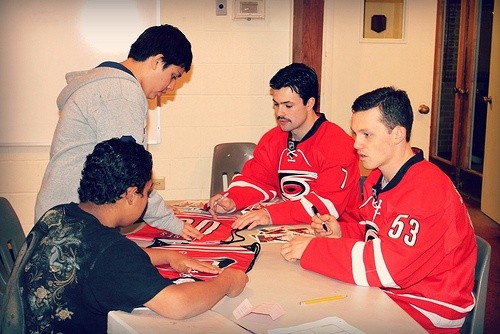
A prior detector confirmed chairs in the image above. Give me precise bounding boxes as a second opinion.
[460,235,491,334]
[210,142,257,199]
[0,197,27,305]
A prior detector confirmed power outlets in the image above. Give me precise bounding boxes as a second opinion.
[154,176,165,190]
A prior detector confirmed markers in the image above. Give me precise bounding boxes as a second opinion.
[311,206,329,232]
[209,192,230,212]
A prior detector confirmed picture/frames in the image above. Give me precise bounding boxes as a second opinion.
[358,0,408,43]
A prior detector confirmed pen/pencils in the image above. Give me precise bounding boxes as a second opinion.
[299,295,349,306]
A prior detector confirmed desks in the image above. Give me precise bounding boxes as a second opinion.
[109,189,429,334]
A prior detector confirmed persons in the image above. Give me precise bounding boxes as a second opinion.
[208,63,363,230]
[281,86,479,334]
[34,24,204,242]
[0,138,248,334]
